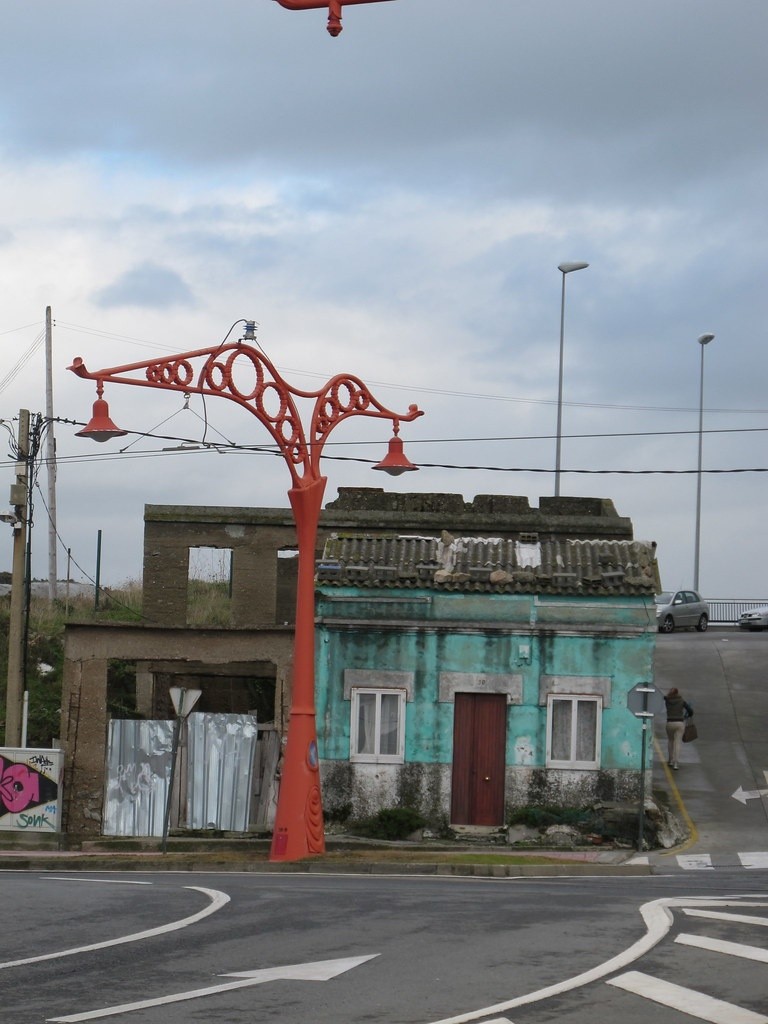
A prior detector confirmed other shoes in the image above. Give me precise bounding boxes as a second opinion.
[673,763,679,770]
[668,760,673,766]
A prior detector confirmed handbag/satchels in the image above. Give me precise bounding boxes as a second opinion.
[682,715,698,742]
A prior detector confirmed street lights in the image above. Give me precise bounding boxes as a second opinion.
[554,258,591,498]
[695,329,718,587]
[66,335,423,861]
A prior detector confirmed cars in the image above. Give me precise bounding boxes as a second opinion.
[736,606,768,634]
[652,590,710,631]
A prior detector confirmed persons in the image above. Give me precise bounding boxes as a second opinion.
[664,687,694,770]
